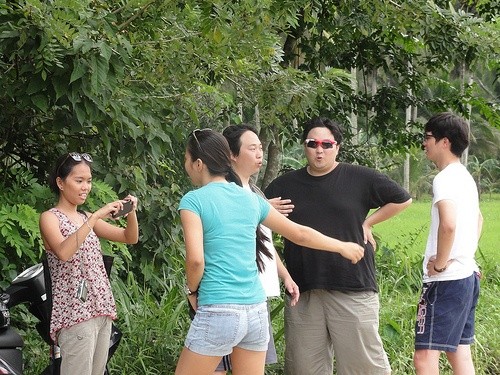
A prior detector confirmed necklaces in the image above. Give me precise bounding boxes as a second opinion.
[60,208,83,228]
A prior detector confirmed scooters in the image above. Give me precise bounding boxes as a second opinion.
[0,261,123,375]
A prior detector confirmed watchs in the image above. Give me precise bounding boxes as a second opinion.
[184,286,197,295]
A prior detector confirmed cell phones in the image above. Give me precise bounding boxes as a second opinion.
[111,200,133,218]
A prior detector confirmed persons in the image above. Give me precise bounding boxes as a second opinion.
[174,128,365,375]
[414,112,483,375]
[40,152,139,375]
[263,117,412,375]
[214,125,300,375]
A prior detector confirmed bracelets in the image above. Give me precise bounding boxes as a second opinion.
[434,264,446,273]
[87,222,91,230]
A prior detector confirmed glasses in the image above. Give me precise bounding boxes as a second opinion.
[57,152,93,178]
[305,139,337,149]
[423,133,435,140]
[189,128,213,154]
